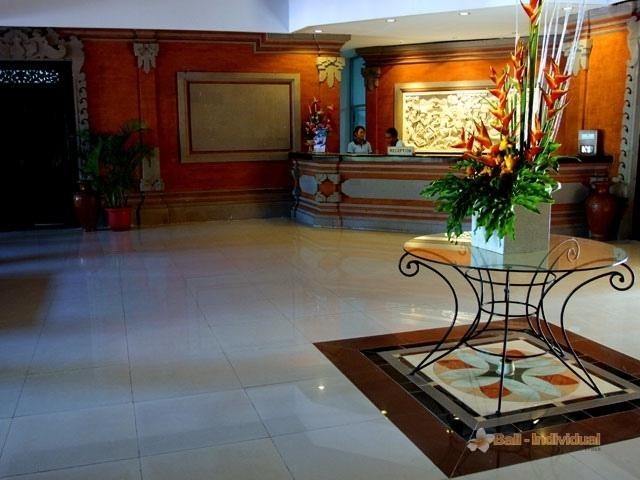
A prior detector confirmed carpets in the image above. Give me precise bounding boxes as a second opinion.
[311,315,639,477]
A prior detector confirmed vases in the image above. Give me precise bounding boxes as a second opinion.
[587,182,623,238]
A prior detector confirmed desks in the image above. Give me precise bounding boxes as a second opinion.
[397,234,633,415]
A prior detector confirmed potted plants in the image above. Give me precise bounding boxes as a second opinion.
[74,120,156,232]
[422,0,586,257]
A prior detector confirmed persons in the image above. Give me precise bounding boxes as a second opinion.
[384,128,406,154]
[348,126,373,154]
